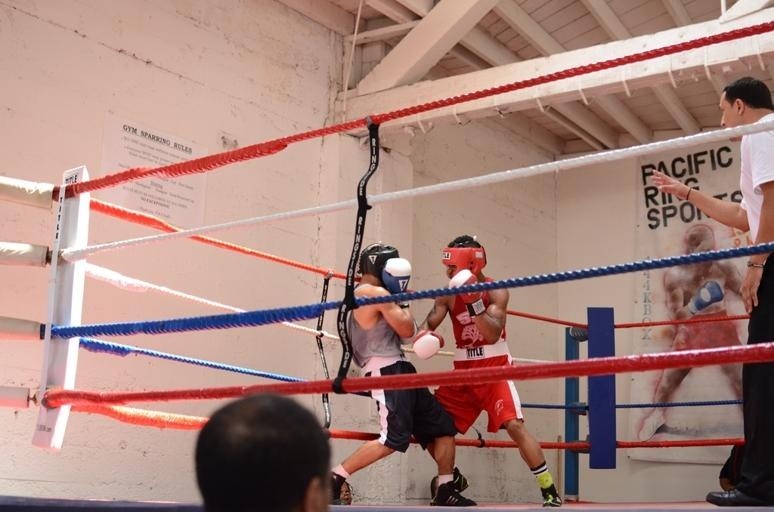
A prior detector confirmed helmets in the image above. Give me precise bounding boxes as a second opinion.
[441,235,486,278]
[360,244,399,276]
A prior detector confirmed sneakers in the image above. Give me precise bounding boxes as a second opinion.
[430,467,468,506]
[332,474,345,504]
[436,481,476,506]
[543,494,561,507]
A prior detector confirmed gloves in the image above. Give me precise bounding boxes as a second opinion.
[383,257,411,307]
[688,281,723,315]
[450,269,489,315]
[412,331,445,359]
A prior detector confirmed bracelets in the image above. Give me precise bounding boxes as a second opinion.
[748,262,765,269]
[685,186,692,202]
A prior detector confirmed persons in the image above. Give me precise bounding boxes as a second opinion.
[330,243,477,506]
[411,236,563,507]
[650,76,773,507]
[637,224,747,443]
[340,481,353,506]
[430,474,440,499]
[194,393,334,512]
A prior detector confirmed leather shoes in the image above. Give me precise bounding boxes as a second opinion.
[706,488,772,506]
